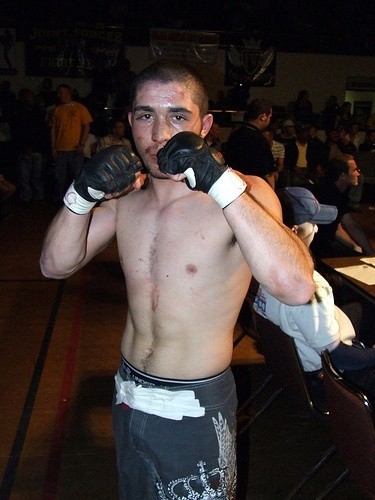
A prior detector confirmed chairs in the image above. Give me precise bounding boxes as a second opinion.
[277,347,375,500]
[233,296,260,349]
[235,319,330,440]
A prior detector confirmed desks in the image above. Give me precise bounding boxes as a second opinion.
[320,255,375,347]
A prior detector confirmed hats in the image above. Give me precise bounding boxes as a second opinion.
[275,186,339,227]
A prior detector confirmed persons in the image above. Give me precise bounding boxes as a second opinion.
[221,98,277,191]
[39,59,319,500]
[10,88,45,205]
[51,84,93,209]
[261,89,375,266]
[252,186,375,409]
[0,80,81,194]
[84,60,139,158]
[204,124,223,153]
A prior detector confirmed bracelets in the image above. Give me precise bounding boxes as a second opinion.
[79,142,85,147]
